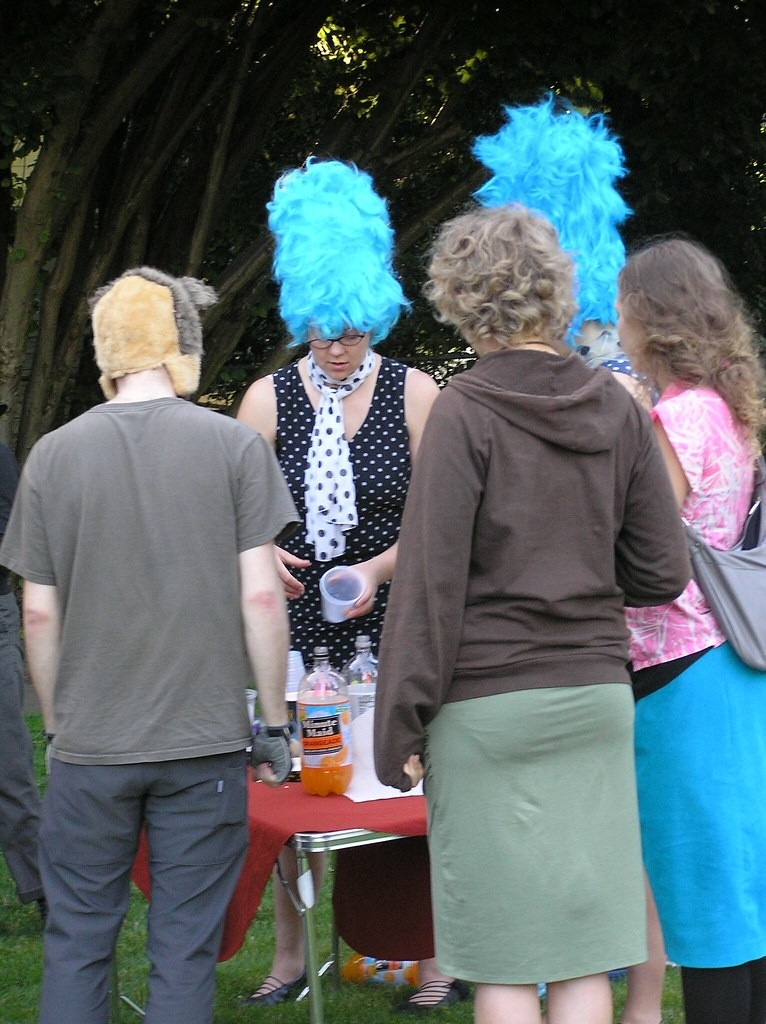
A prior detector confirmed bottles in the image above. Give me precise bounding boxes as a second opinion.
[296,646,354,796]
[329,952,420,988]
[341,635,379,724]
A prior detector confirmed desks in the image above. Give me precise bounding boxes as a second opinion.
[127,733,454,1023]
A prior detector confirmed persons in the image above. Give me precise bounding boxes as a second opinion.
[613,238,766,1024]
[1,265,304,1024]
[237,326,470,1013]
[373,205,693,1024]
[571,316,661,412]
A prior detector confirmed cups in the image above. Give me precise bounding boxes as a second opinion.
[285,650,306,702]
[318,566,367,624]
[244,689,258,725]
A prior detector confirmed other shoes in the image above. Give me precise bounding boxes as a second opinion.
[243,968,308,1004]
[36,896,47,920]
[395,979,470,1012]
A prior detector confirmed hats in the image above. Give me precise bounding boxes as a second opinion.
[0,404,9,418]
[88,266,220,401]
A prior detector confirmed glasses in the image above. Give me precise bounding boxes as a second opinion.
[306,332,365,350]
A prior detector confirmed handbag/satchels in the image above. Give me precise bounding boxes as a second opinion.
[680,454,766,671]
